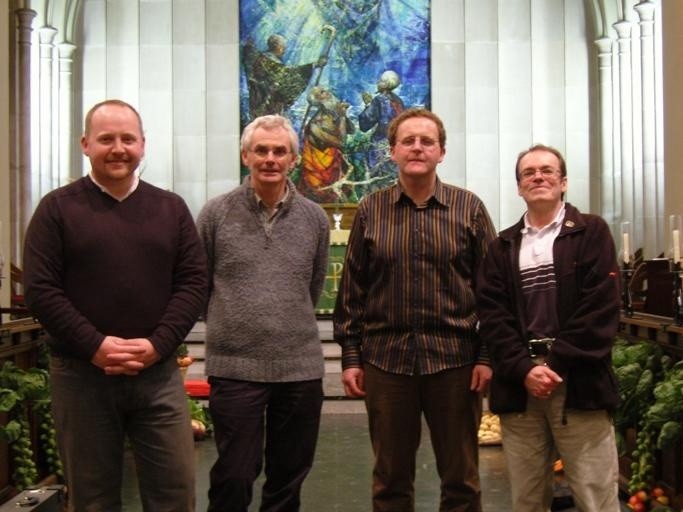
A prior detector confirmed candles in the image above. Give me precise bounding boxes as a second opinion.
[623,233,629,263]
[673,230,681,264]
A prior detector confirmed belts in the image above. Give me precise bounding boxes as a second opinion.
[529,337,559,360]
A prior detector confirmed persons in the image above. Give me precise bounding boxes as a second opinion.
[300,86,355,203]
[194,112,330,512]
[359,70,406,193]
[333,108,499,512]
[22,99,213,512]
[244,32,328,122]
[477,146,622,512]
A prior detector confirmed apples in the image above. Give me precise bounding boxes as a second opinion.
[629,487,669,511]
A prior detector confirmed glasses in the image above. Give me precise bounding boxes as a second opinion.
[518,166,562,179]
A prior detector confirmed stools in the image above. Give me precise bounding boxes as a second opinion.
[184,380,210,437]
